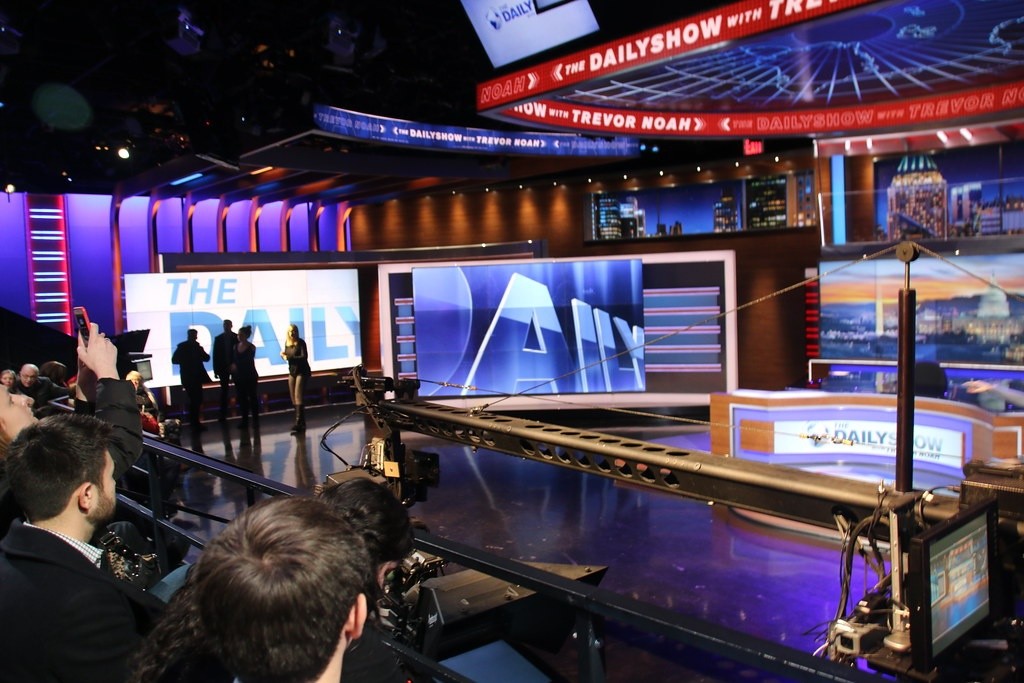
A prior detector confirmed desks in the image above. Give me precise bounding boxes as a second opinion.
[867,616,1024,683]
[710,390,1023,479]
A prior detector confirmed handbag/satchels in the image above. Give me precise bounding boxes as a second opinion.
[100,534,162,591]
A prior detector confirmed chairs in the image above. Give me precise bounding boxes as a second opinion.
[911,361,948,399]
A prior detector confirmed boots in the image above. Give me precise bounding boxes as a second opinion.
[290,404,306,432]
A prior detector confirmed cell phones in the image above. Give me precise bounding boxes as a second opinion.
[73,306,91,347]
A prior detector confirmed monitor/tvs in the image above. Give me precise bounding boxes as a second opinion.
[905,496,999,675]
[457,0,601,68]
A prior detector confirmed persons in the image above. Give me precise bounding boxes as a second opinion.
[0,323,165,591]
[227,325,260,430]
[0,362,182,445]
[192,498,374,683]
[128,476,416,683]
[172,330,211,432]
[213,320,239,421]
[280,324,312,432]
[0,415,170,683]
